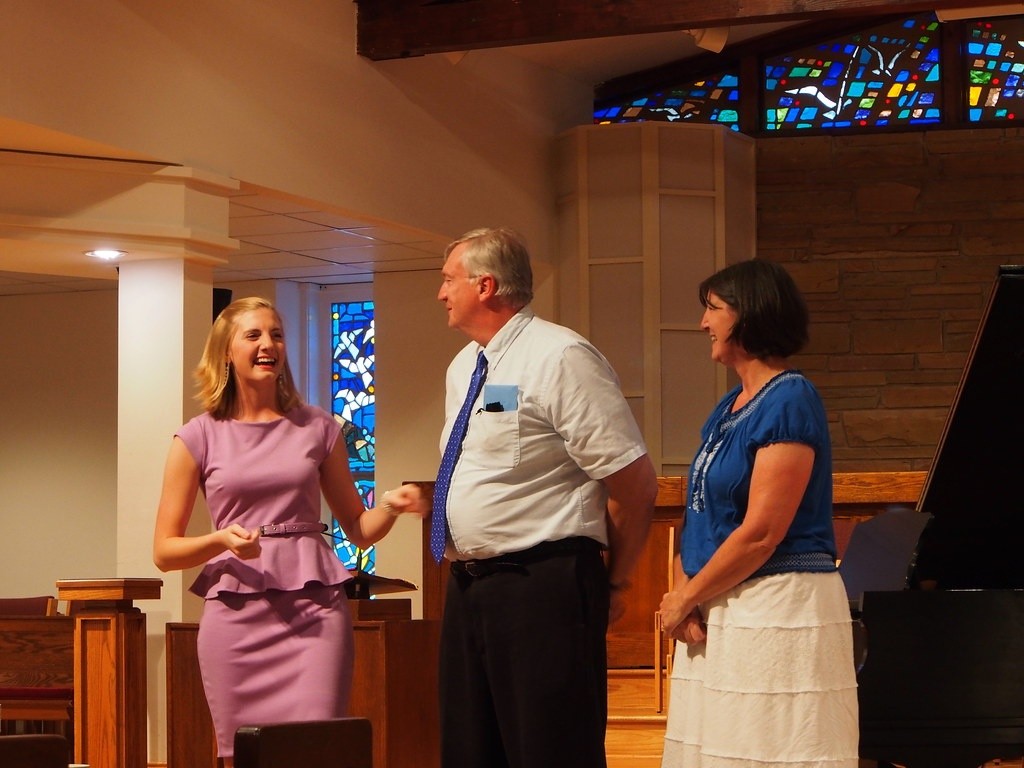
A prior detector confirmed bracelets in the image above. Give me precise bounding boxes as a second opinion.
[380,491,401,518]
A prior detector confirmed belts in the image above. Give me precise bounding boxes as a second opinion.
[448,536,599,584]
[247,523,328,537]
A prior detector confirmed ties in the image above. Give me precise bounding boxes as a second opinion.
[432,352,489,564]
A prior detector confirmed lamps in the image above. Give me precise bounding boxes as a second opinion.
[683,26,730,54]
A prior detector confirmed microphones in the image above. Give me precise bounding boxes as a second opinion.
[321,523,367,573]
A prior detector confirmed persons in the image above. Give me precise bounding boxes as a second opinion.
[660,255,861,768]
[153,297,433,768]
[435,227,658,768]
